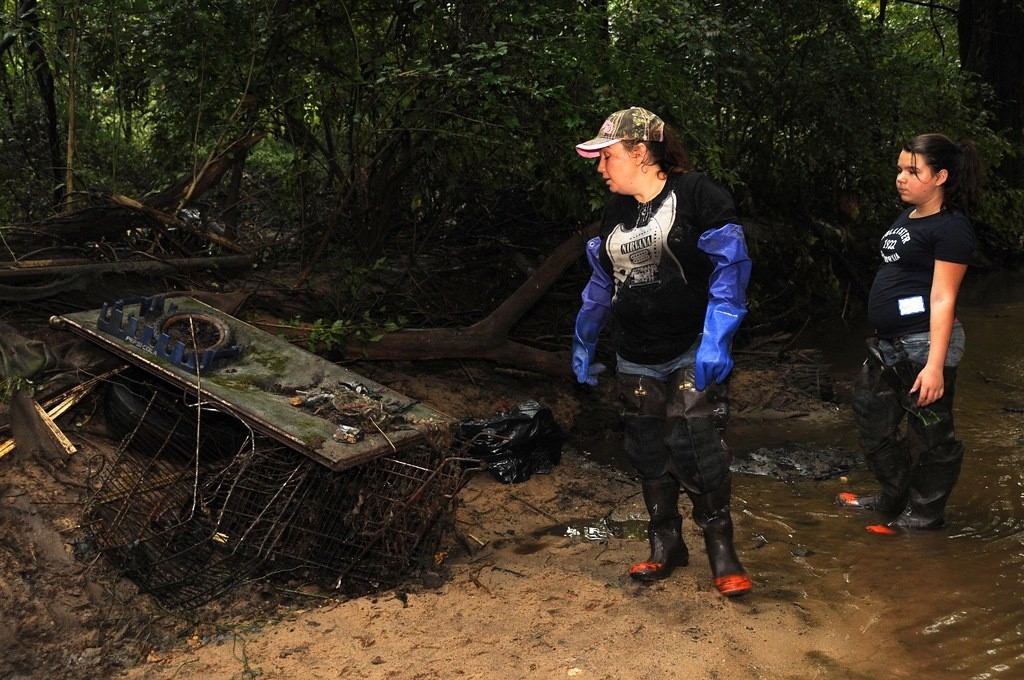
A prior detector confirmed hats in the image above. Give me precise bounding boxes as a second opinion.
[575,106,665,158]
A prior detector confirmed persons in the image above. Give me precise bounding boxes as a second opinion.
[570,105,751,598]
[834,133,975,535]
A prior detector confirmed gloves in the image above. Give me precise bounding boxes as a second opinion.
[695,223,752,392]
[571,235,612,387]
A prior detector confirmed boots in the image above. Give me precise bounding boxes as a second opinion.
[630,480,689,582]
[692,485,752,597]
[835,432,913,515]
[864,440,964,535]
